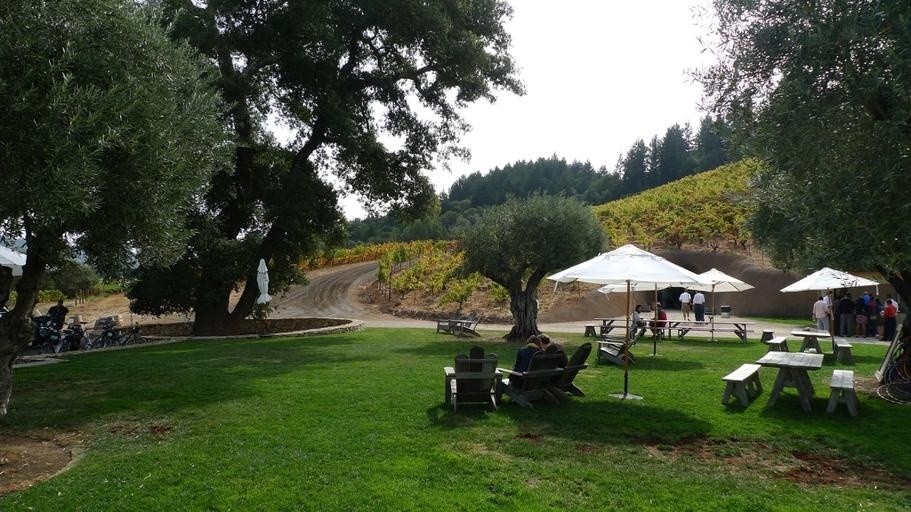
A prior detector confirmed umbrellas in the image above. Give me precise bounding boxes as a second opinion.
[256,258,272,334]
[779,266,880,294]
[597,282,671,319]
[683,267,756,329]
[545,244,699,340]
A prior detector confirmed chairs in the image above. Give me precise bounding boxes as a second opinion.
[434,310,485,338]
[595,323,646,366]
[496,350,565,409]
[554,343,592,396]
[442,353,503,413]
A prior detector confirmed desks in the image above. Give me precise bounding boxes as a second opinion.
[594,318,756,338]
[756,351,824,411]
[791,328,831,353]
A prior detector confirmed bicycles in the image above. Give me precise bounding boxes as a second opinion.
[31,317,150,357]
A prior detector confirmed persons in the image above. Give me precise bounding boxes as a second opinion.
[649,302,667,337]
[633,305,647,336]
[813,291,899,342]
[679,289,691,322]
[537,334,568,368]
[693,291,705,322]
[48,299,69,328]
[509,335,543,383]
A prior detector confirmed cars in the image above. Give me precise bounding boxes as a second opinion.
[0,306,11,318]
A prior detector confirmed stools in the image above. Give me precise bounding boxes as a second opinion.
[760,330,775,344]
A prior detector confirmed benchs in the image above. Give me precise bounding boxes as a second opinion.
[766,336,788,352]
[584,324,754,343]
[721,364,762,406]
[826,370,859,416]
[832,336,854,362]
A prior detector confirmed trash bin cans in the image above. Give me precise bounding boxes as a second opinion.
[720,305,732,318]
